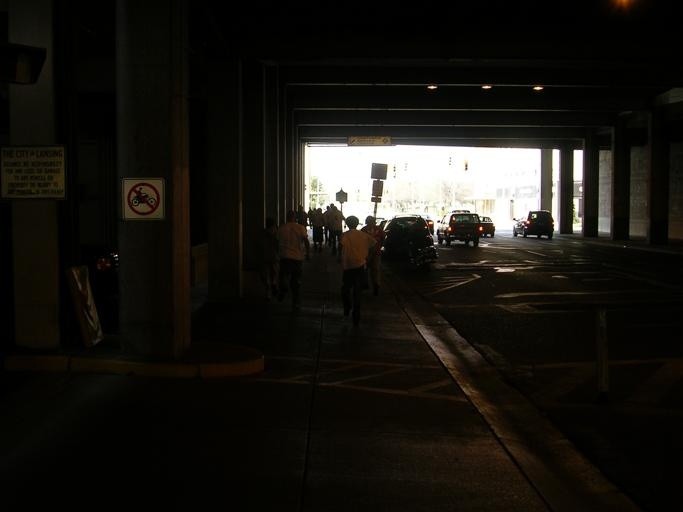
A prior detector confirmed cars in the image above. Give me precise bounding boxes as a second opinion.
[479,216,495,238]
[376,216,438,265]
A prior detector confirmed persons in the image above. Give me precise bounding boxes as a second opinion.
[275,204,387,329]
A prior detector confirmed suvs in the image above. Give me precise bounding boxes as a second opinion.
[437,209,481,247]
[513,210,554,240]
